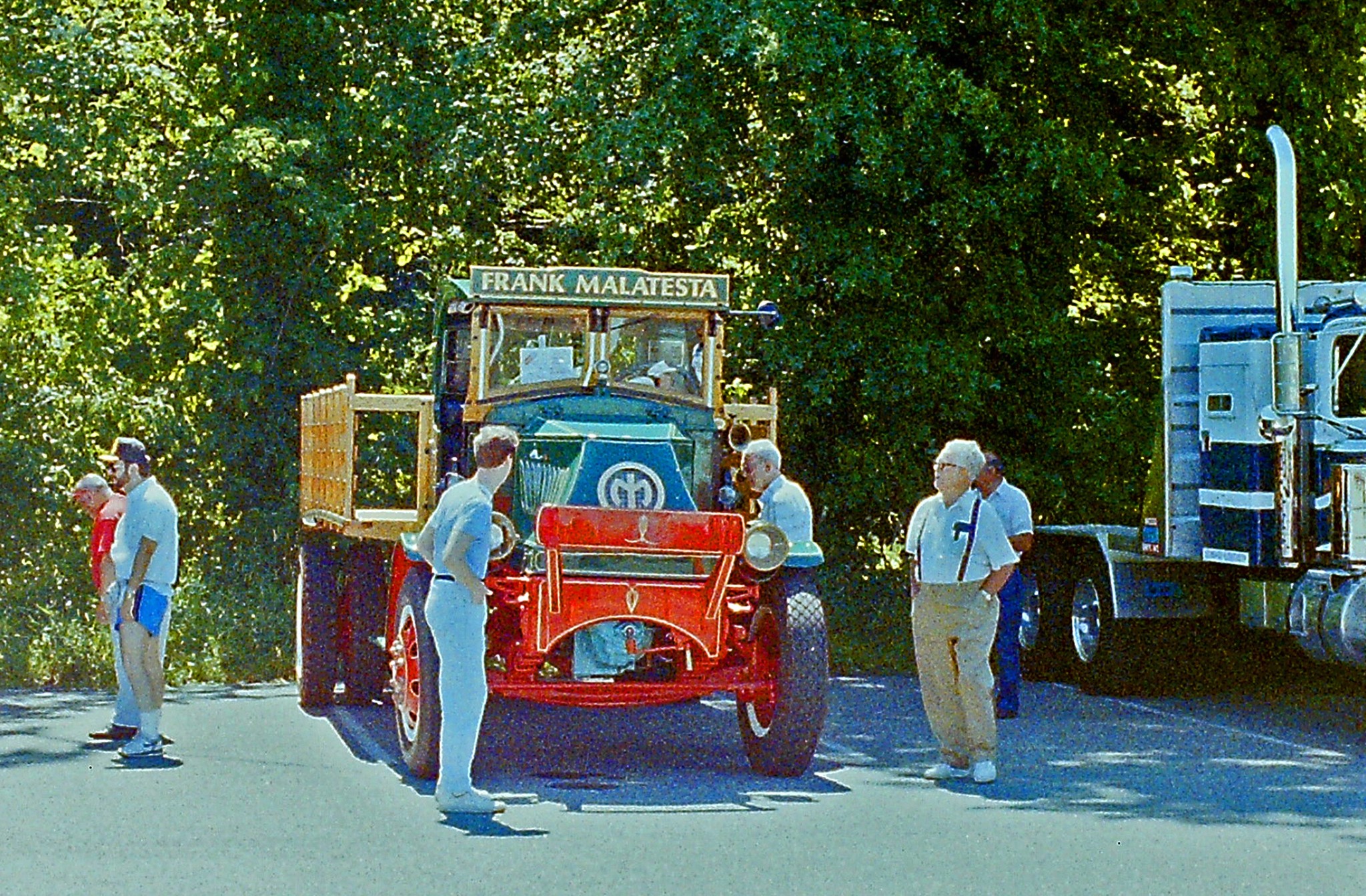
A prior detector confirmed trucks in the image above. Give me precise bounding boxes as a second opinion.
[289,263,832,782]
[1018,122,1365,698]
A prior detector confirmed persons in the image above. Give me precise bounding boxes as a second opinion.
[414,424,518,815]
[740,438,813,544]
[972,451,1033,720]
[75,473,172,742]
[905,439,1021,784]
[100,436,179,759]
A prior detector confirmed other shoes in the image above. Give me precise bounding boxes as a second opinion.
[118,736,164,759]
[924,763,970,779]
[973,761,997,782]
[437,788,506,814]
[89,723,136,740]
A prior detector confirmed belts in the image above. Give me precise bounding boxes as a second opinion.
[434,575,454,582]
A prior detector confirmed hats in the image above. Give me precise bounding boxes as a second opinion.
[98,437,148,464]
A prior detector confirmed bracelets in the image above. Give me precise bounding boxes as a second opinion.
[124,591,135,600]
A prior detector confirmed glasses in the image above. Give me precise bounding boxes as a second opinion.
[933,462,955,471]
[111,464,120,472]
